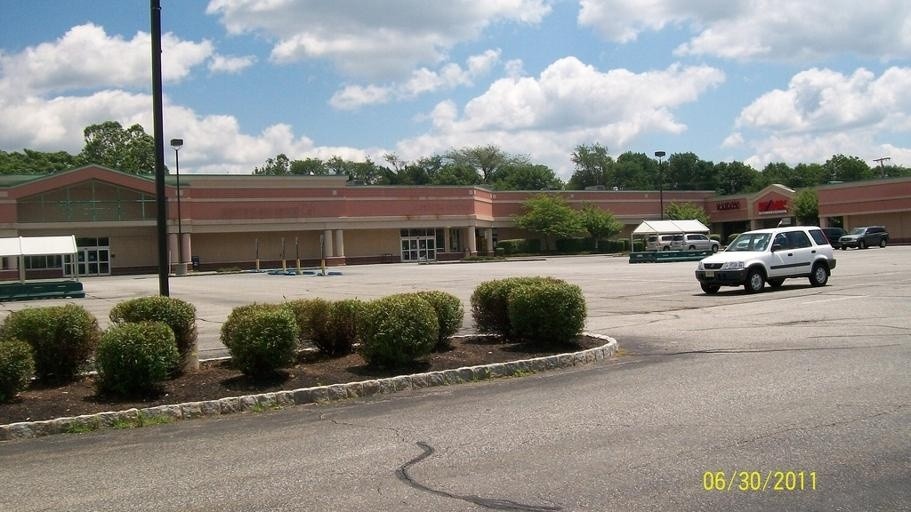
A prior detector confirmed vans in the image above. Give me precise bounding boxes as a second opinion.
[645,233,671,252]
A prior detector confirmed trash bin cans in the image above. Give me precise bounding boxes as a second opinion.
[496,247,504,257]
[464,247,471,257]
[384,253,392,263]
[192,256,199,270]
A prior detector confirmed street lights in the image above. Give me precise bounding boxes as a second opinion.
[171,139,184,264]
[656,151,666,220]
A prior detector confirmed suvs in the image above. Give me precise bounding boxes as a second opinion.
[669,233,722,253]
[696,226,837,293]
[839,225,889,249]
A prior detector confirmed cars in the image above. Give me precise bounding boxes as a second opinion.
[821,227,848,249]
